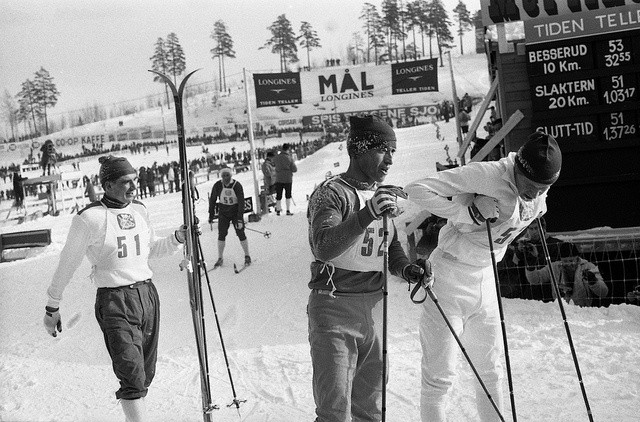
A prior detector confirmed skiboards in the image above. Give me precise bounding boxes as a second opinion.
[148,67,217,420]
[199,263,246,278]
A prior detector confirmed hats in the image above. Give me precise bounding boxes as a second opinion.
[98,154,136,181]
[221,165,232,177]
[515,131,562,184]
[560,242,579,258]
[348,116,397,158]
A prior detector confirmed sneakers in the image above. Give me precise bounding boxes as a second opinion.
[216,257,223,265]
[245,256,251,265]
[277,211,280,215]
[287,210,293,215]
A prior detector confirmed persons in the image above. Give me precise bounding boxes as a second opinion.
[307,116,435,422]
[207,167,251,269]
[519,245,610,310]
[404,132,563,422]
[84,163,181,204]
[437,89,505,169]
[262,150,276,206]
[11,166,28,209]
[43,154,201,421]
[514,219,578,264]
[276,143,296,217]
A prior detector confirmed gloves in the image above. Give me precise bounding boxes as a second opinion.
[365,184,408,219]
[525,251,537,271]
[402,258,435,289]
[235,220,243,229]
[582,269,598,285]
[468,194,500,226]
[44,306,62,336]
[175,216,203,244]
[209,215,214,223]
